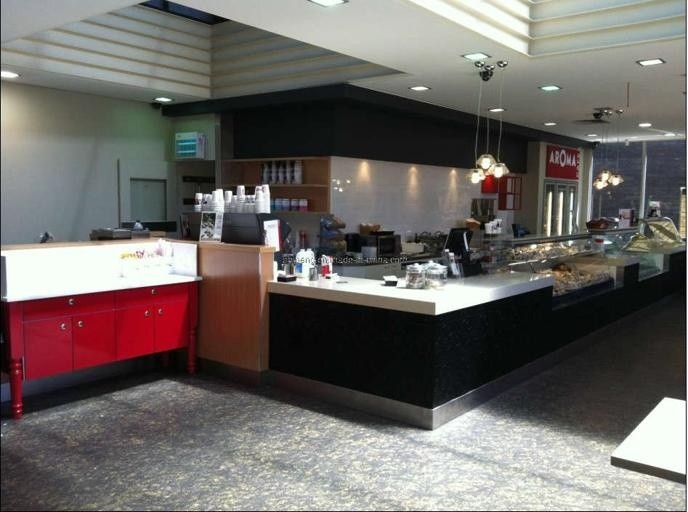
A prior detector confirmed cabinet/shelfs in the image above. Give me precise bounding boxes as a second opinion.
[0,283,203,419]
[221,156,331,215]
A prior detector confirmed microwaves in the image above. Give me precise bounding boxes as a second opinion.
[367,235,401,260]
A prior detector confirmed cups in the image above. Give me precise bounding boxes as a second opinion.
[270,198,309,213]
[321,264,333,279]
[194,183,270,214]
[485,217,503,233]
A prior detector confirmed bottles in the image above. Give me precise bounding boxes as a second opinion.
[405,259,449,289]
[295,248,315,278]
[133,220,142,230]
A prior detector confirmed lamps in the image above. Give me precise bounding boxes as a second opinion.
[592,107,624,191]
[466,60,509,184]
[174,130,205,161]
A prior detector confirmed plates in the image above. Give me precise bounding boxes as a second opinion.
[354,246,377,260]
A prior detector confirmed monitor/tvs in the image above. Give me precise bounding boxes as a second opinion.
[441,227,473,257]
